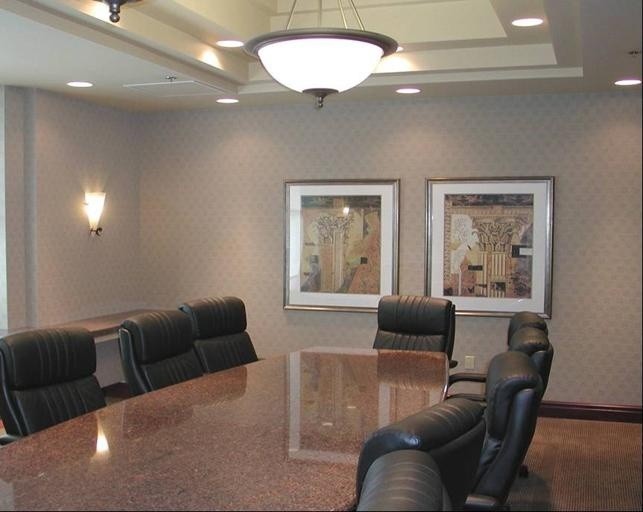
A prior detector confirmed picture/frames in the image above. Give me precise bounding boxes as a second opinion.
[424,176,552,320]
[282,178,399,313]
[285,346,391,468]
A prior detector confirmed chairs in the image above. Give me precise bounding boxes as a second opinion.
[177,296,258,375]
[358,398,487,511]
[117,311,204,398]
[506,325,554,390]
[449,349,546,511]
[444,310,549,401]
[370,297,459,371]
[0,326,107,444]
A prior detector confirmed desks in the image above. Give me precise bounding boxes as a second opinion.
[0,349,450,511]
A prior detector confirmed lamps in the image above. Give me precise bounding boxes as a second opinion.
[83,191,106,236]
[94,1,141,22]
[245,0,399,110]
